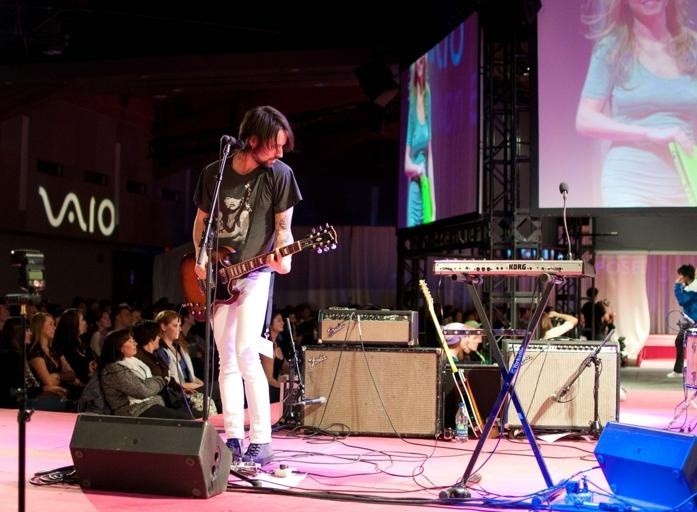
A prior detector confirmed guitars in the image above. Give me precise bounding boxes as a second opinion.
[180,222,338,322]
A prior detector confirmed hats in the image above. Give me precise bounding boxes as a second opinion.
[443,319,483,347]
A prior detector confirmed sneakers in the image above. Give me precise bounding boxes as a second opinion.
[242,443,274,465]
[223,439,243,461]
[666,371,683,379]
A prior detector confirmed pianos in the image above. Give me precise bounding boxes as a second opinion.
[432,257,596,278]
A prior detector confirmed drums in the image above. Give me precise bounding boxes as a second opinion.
[683,328,697,389]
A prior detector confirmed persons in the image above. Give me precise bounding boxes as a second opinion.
[571,0,696,208]
[667,262,696,381]
[349,285,614,421]
[400,51,439,230]
[192,105,303,469]
[2,296,318,423]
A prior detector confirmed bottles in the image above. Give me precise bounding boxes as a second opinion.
[455,403,469,443]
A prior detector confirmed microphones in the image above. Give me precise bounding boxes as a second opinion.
[559,182,574,261]
[222,134,245,150]
[299,397,326,406]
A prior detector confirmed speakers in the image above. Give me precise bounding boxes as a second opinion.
[502,340,618,436]
[70,413,233,498]
[445,365,500,437]
[319,312,418,346]
[301,345,441,439]
[595,421,697,510]
